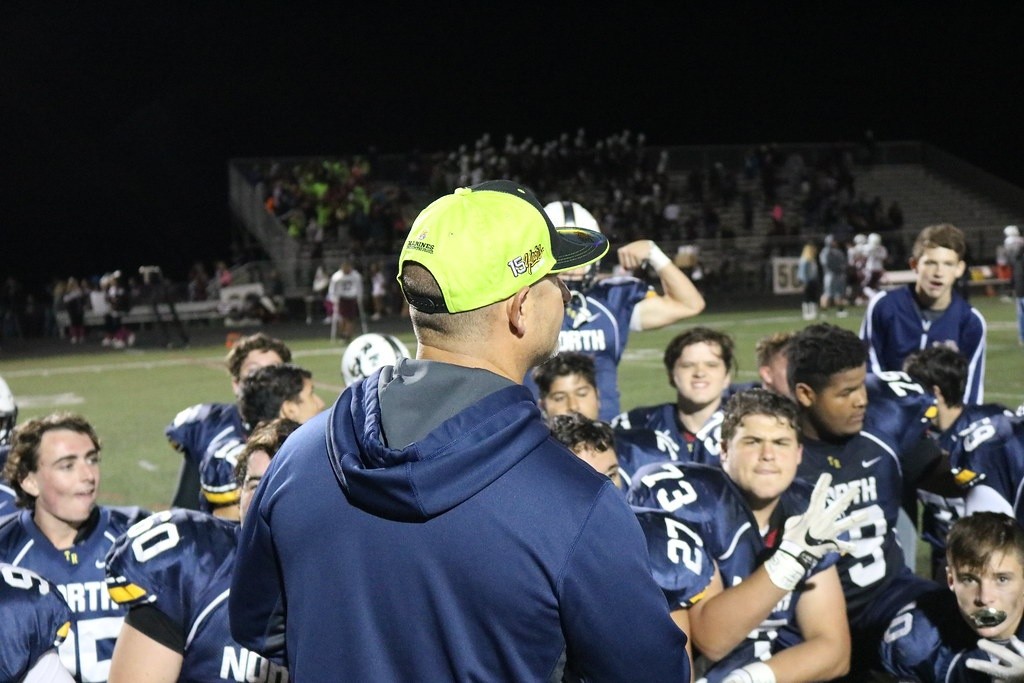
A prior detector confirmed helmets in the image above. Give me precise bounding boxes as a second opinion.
[542,198,602,297]
[0,376,17,452]
[824,235,839,247]
[869,233,882,244]
[855,235,867,245]
[340,333,412,390]
[1004,226,1020,236]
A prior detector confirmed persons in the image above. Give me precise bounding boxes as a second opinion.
[0,180,1024,683]
[0,127,1024,348]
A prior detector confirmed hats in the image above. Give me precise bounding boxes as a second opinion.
[397,178,610,315]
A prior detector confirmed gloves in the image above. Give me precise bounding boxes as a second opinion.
[764,470,867,587]
[966,635,1024,683]
